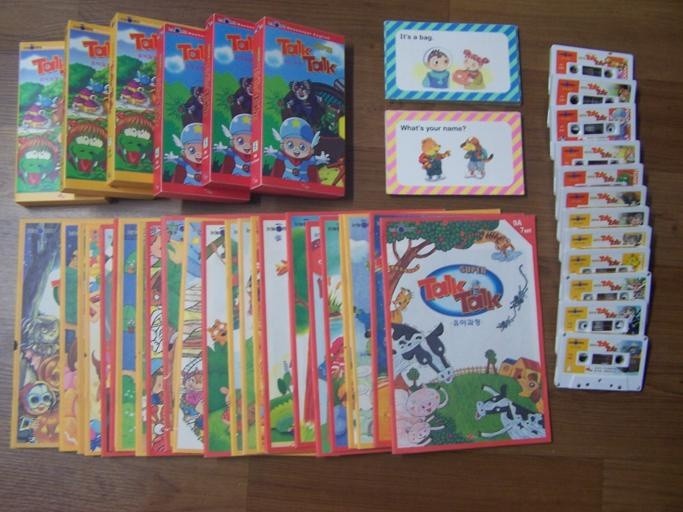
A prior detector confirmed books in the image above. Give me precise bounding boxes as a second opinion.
[11,208,554,461]
